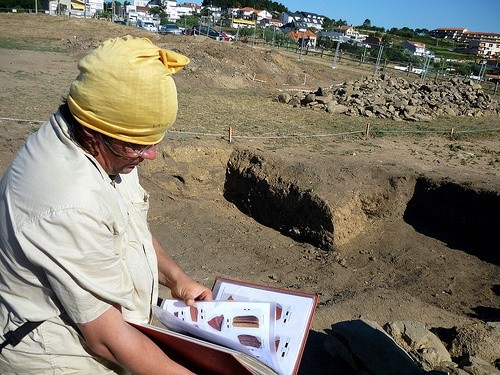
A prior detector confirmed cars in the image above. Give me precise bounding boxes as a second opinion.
[190,25,220,42]
[395,63,410,71]
[220,32,235,41]
[160,24,183,35]
[412,66,426,75]
[136,19,158,32]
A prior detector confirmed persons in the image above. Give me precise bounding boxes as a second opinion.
[0,34,215,375]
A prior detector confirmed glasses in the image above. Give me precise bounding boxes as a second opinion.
[128,143,153,158]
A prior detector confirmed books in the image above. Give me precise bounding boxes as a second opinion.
[122,275,318,375]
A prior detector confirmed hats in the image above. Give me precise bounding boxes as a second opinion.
[67,35,190,145]
